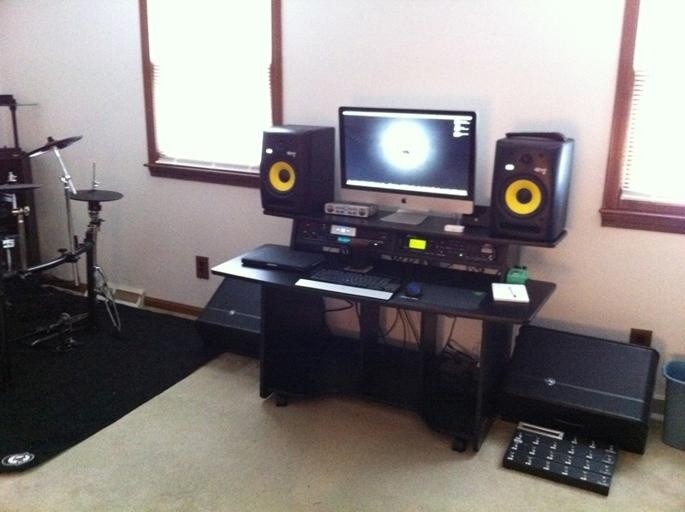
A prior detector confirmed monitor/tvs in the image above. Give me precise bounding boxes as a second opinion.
[338,106,476,226]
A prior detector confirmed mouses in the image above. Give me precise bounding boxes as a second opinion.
[405,280,423,296]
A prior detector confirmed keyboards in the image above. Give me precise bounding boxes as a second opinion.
[294,268,402,301]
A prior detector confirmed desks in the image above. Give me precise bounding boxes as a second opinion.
[210,211,555,450]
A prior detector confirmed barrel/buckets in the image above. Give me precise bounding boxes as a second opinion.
[660,361,685,449]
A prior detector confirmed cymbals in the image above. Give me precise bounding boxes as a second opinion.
[70,189,123,201]
[26,136,83,157]
[0,184,43,189]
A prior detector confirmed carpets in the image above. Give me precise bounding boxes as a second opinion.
[0,284,236,475]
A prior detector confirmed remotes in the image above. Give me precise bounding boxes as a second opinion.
[507,131,566,142]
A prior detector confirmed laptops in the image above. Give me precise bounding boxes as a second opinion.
[240,243,324,274]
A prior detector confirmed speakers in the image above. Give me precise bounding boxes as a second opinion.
[193,276,263,359]
[498,324,659,456]
[259,124,335,216]
[486,138,575,240]
